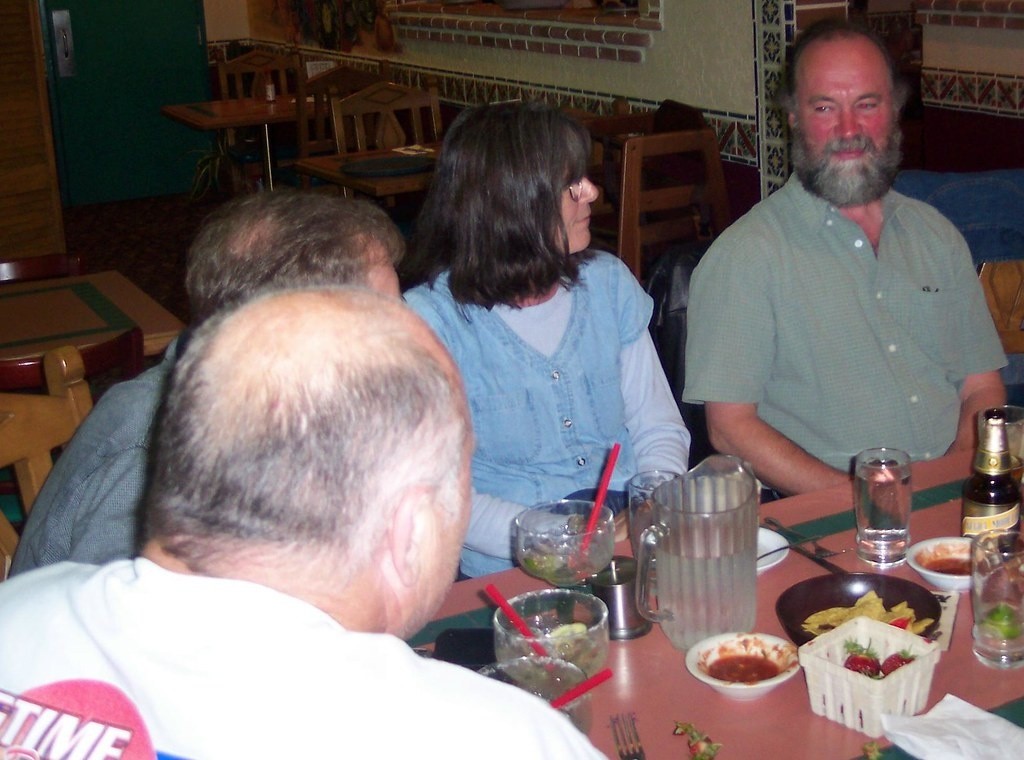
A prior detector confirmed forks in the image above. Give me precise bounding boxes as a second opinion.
[610,712,647,760]
[765,517,842,560]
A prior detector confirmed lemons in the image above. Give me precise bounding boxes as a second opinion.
[981,605,1023,638]
[547,623,588,641]
[524,555,561,575]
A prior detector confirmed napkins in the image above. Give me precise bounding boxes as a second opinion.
[879,691,1024,760]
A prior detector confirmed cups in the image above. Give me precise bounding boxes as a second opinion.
[514,498,616,588]
[474,654,593,736]
[969,529,1024,671]
[978,404,1024,459]
[627,468,683,559]
[854,448,912,570]
[589,567,652,640]
[492,587,611,688]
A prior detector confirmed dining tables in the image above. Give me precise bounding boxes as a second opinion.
[407,445,1024,760]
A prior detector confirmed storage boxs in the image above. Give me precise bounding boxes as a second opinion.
[799,614,941,741]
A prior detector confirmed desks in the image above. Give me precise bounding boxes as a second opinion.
[0,270,186,362]
[160,93,334,195]
[290,138,444,207]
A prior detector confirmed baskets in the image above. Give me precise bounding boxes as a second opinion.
[798,614,943,737]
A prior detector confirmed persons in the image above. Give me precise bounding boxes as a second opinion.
[6,191,407,578]
[680,18,1010,503]
[0,284,611,760]
[400,102,691,579]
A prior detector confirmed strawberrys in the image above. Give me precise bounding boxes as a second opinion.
[880,650,915,676]
[845,649,879,677]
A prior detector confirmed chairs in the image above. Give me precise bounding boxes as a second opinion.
[0,254,84,285]
[0,346,94,582]
[0,325,145,536]
[589,98,731,281]
[296,60,385,191]
[216,51,309,194]
[331,79,443,210]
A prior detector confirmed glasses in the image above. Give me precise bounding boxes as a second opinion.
[561,179,584,201]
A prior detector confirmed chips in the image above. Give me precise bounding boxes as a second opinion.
[800,589,935,636]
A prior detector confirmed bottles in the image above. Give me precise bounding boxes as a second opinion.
[959,407,1022,562]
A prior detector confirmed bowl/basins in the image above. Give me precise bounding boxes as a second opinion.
[756,527,789,576]
[775,572,942,647]
[685,632,801,702]
[906,535,1000,593]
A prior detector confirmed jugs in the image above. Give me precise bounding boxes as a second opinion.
[634,454,759,652]
